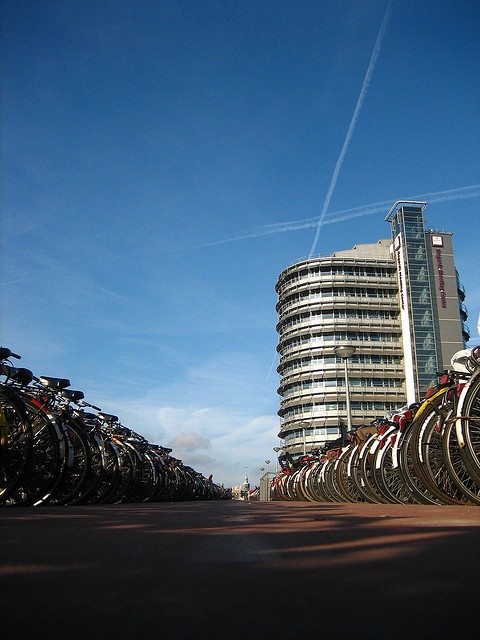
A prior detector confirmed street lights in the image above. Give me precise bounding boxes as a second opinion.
[260,467,265,474]
[265,460,271,473]
[298,421,309,454]
[332,345,356,433]
[273,446,280,474]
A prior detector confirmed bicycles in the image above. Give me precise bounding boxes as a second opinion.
[356,344,480,506]
[241,486,260,501]
[279,430,356,503]
[270,474,279,501]
[0,345,232,508]
